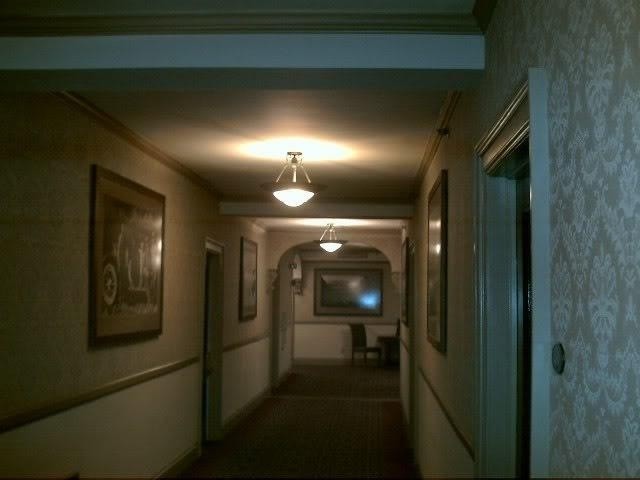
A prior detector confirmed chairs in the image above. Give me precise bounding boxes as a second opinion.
[349,324,381,367]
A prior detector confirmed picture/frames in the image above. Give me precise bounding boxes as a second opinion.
[400,238,409,327]
[313,268,384,316]
[427,169,447,354]
[240,236,257,321]
[90,165,165,348]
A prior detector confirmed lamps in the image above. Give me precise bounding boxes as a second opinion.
[314,224,348,253]
[260,152,327,207]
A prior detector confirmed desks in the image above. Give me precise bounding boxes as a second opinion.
[377,336,399,369]
